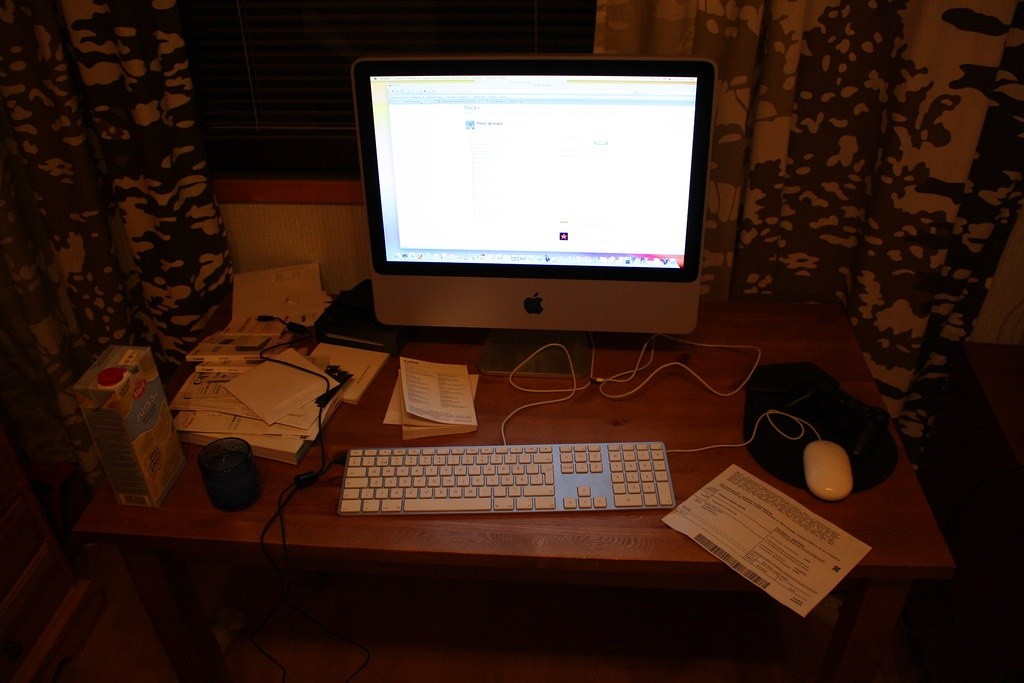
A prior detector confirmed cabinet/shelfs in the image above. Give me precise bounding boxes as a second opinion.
[0,413,104,683]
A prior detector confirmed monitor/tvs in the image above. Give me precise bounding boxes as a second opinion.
[351,54,718,377]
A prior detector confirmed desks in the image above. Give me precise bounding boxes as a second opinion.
[72,303,954,683]
[902,341,1024,683]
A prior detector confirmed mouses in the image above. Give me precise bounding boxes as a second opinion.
[803,440,853,501]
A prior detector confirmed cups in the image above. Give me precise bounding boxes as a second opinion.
[197,437,259,513]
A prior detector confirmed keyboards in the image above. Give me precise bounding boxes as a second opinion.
[338,441,675,515]
